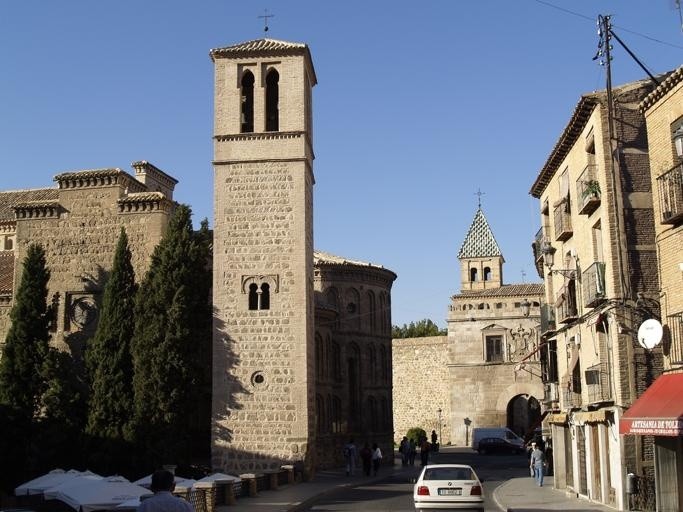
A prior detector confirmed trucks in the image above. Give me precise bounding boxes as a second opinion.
[471,426,523,452]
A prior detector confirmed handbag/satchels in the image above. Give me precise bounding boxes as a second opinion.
[530,467,535,477]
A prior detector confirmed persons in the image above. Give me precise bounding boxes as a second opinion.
[136,469,196,512]
[131,472,239,492]
[338,429,439,477]
[530,440,546,488]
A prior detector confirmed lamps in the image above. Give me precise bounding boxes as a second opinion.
[540,239,582,283]
[520,297,544,326]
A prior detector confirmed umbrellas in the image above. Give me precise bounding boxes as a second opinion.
[13,461,152,510]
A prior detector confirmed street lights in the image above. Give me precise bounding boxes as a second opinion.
[436,407,442,447]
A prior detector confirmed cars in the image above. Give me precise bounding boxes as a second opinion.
[411,464,484,512]
[478,436,520,455]
